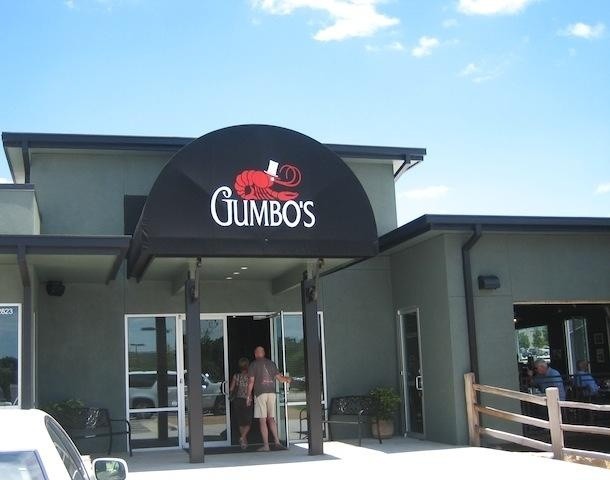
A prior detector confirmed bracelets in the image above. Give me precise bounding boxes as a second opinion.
[247,397,251,399]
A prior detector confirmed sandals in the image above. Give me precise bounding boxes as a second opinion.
[238,436,248,450]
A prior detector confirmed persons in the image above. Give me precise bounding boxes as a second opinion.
[230,357,254,449]
[574,359,599,394]
[525,359,566,431]
[246,345,292,452]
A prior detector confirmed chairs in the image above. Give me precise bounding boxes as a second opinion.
[569,384,602,440]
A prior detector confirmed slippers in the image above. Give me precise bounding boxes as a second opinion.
[255,447,270,452]
[274,443,286,450]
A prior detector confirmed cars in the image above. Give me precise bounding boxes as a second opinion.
[279,378,307,402]
[0,408,128,480]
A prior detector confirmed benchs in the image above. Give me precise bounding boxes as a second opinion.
[55,407,133,457]
[321,395,383,446]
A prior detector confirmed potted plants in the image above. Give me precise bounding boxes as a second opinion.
[368,384,402,439]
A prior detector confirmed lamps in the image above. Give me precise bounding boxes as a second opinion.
[46,281,65,297]
[477,275,500,290]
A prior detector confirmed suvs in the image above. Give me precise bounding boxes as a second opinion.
[129,371,226,419]
